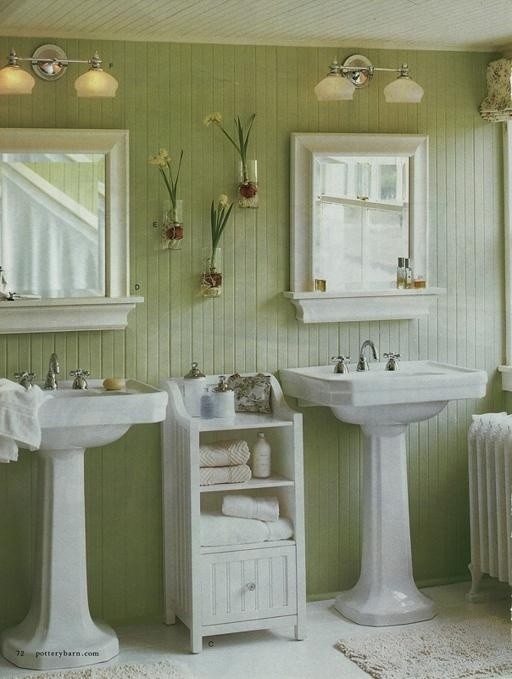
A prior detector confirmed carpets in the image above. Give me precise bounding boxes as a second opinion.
[8,659,193,679]
[336,612,512,679]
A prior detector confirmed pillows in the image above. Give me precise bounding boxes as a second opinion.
[200,511,294,547]
[0,378,53,464]
[222,494,279,521]
[199,439,250,467]
[200,464,251,486]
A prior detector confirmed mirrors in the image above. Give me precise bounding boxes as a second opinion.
[0,127,144,334]
[283,132,446,323]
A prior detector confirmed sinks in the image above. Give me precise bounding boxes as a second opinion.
[278,359,488,424]
[34,378,169,451]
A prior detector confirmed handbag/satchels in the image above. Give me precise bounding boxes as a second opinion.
[226,374,271,413]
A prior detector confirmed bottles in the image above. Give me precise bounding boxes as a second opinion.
[396,255,413,290]
[250,431,273,479]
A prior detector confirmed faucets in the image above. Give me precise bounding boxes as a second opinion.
[45,353,60,388]
[355,339,378,372]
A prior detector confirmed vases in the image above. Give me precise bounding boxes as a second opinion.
[163,200,183,249]
[201,248,222,298]
[238,161,259,208]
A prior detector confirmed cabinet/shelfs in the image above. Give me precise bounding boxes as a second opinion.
[161,373,306,653]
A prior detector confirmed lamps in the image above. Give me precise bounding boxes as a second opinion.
[314,54,424,103]
[0,43,119,97]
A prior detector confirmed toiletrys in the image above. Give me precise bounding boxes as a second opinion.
[405,257,412,289]
[397,256,405,290]
[413,275,426,289]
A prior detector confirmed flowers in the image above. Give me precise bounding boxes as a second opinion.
[149,147,185,227]
[210,195,234,274]
[203,113,257,185]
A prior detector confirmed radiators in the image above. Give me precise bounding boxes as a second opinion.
[463,411,512,603]
